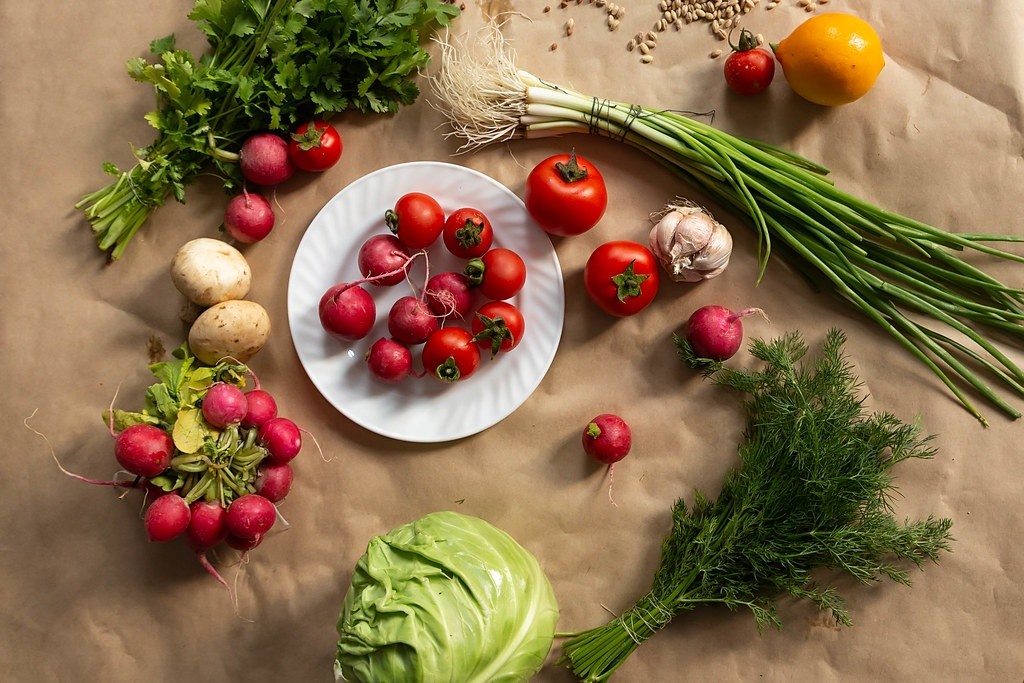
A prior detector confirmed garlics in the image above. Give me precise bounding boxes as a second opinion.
[647,196,733,282]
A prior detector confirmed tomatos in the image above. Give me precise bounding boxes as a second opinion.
[289,117,343,172]
[725,27,776,96]
[393,146,659,380]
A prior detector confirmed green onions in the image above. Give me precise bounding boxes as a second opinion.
[418,11,1024,427]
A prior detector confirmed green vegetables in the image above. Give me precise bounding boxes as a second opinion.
[72,0,459,261]
[555,329,954,683]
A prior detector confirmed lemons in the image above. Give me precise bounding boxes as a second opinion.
[769,14,885,106]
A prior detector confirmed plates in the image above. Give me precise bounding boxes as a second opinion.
[286,160,564,443]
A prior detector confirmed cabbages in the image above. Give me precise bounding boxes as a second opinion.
[335,512,559,682]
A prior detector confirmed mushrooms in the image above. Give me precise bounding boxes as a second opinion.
[169,237,271,363]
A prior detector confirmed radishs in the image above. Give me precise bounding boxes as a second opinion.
[22,353,333,608]
[225,187,274,243]
[320,235,470,383]
[239,132,293,186]
